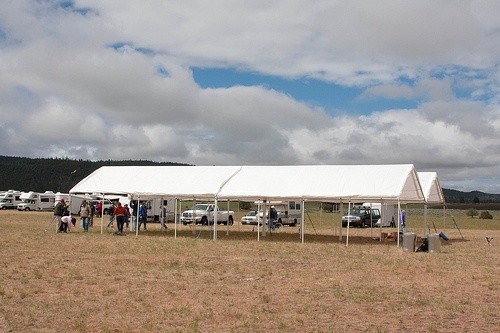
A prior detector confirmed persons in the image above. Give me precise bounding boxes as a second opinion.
[159,206,168,228]
[138,202,152,230]
[106,200,131,234]
[130,200,140,230]
[79,200,102,232]
[54,198,76,233]
[267,206,277,231]
[399,209,406,231]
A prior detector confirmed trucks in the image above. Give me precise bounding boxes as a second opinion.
[0,190,95,216]
[254,200,301,228]
[131,198,175,219]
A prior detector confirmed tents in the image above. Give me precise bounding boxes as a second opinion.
[130,171,445,236]
[69,164,425,248]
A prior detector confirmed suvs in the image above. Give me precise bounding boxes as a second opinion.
[342,206,380,228]
[93,200,115,216]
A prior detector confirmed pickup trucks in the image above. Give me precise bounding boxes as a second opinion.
[180,204,234,226]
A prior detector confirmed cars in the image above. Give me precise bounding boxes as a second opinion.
[241,211,258,225]
